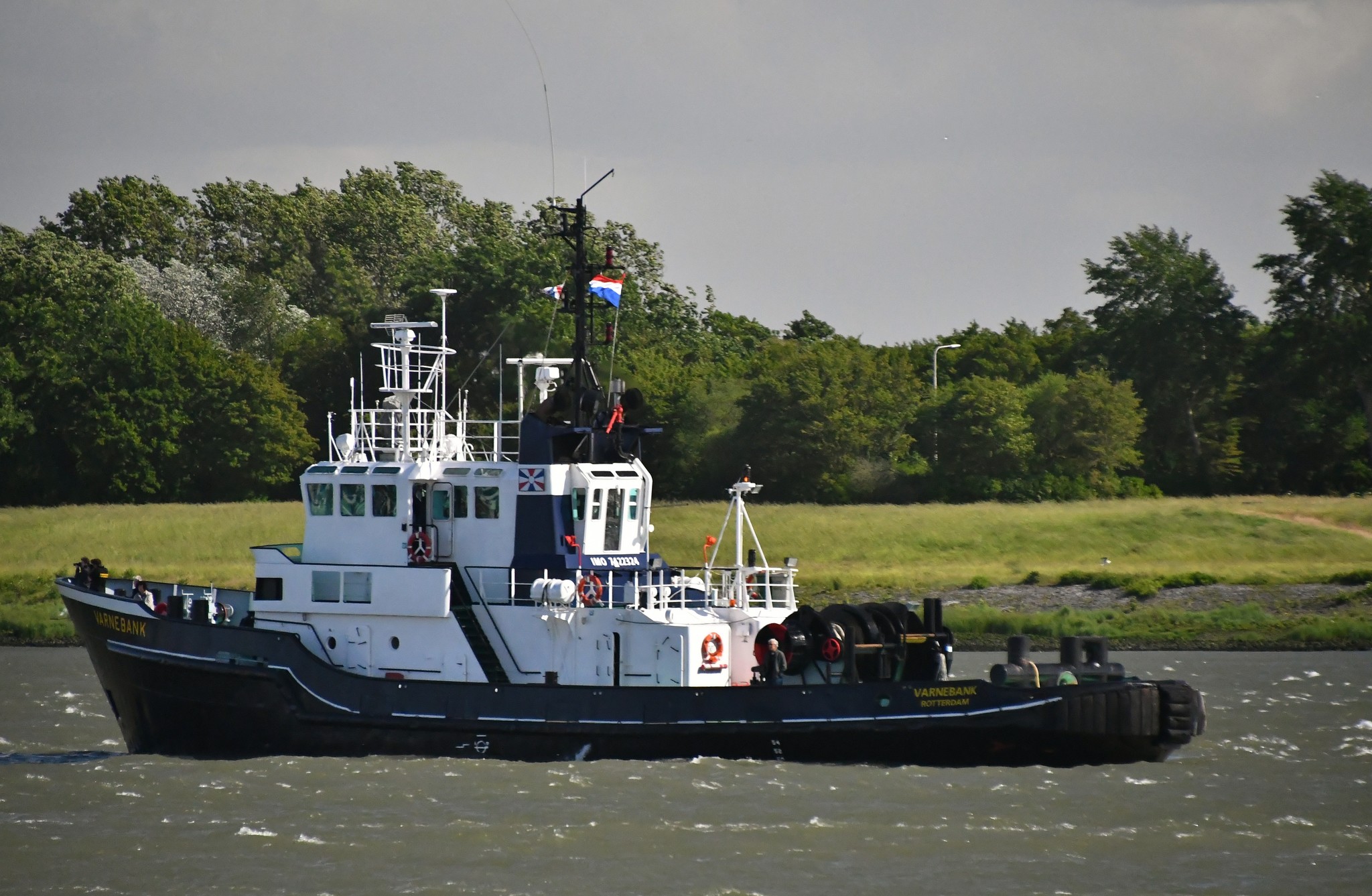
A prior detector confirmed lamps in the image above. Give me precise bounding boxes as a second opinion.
[751,485,764,494]
[741,463,752,482]
[784,557,798,568]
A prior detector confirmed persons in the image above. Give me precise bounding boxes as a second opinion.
[133,581,154,612]
[762,638,786,685]
[75,557,108,593]
[129,575,142,599]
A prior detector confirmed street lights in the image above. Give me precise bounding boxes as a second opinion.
[933,344,961,464]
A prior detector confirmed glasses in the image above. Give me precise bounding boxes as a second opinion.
[768,643,775,645]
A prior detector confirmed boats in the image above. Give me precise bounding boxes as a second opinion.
[51,163,1205,772]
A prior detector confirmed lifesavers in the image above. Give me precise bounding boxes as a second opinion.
[701,632,723,663]
[407,531,432,563]
[578,574,603,605]
[746,570,766,599]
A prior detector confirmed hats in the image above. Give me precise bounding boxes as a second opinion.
[135,576,142,581]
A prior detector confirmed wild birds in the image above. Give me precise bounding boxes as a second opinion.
[1098,556,1112,567]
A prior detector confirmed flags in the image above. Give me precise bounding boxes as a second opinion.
[589,274,622,308]
[540,284,564,300]
